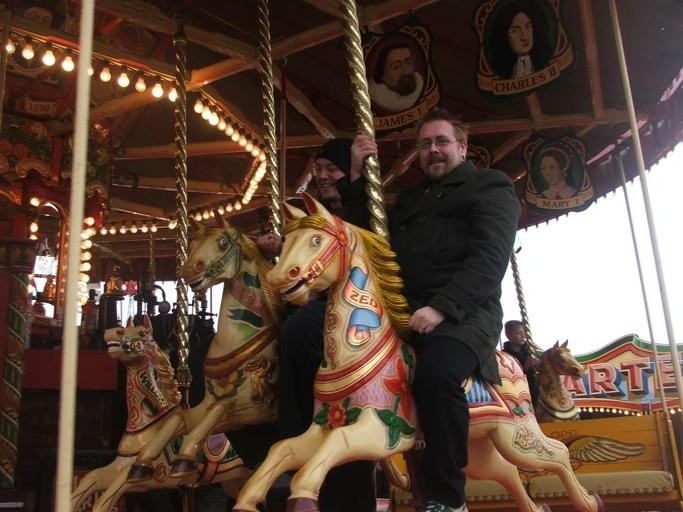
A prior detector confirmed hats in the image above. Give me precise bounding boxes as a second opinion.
[313,138,353,175]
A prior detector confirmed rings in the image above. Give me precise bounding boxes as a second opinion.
[267,236,273,239]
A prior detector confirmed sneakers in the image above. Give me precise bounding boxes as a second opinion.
[419,502,468,512]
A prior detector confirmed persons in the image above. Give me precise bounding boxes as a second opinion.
[536,144,581,198]
[153,302,174,348]
[486,2,556,81]
[334,105,524,510]
[366,41,427,119]
[187,314,215,409]
[501,319,542,412]
[255,136,359,438]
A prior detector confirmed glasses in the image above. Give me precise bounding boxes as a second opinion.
[414,140,457,149]
